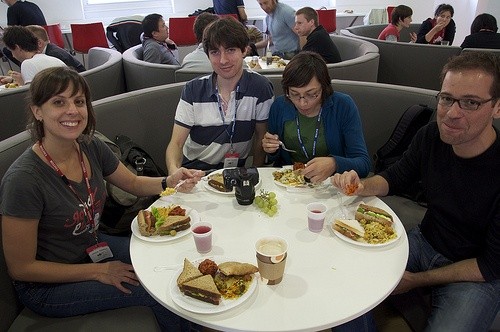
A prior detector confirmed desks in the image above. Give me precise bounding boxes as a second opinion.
[61,29,72,49]
[244,56,291,69]
[130,167,409,332]
[247,14,366,28]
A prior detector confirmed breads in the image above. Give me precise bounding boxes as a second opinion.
[198,259,218,276]
[293,162,305,170]
[218,261,259,276]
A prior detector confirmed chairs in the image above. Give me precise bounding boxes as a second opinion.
[366,8,386,24]
[70,22,115,66]
[45,23,76,56]
[316,9,337,33]
[168,16,197,46]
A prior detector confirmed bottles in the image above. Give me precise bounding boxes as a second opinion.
[252,56,258,65]
[267,49,272,65]
[235,172,256,205]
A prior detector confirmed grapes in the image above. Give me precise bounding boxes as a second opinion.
[255,191,278,216]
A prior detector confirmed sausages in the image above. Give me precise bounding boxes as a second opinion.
[208,179,226,192]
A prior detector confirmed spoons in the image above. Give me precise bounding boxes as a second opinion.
[161,169,201,201]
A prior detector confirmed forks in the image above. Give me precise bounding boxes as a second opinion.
[266,132,296,152]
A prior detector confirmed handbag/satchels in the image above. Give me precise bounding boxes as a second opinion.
[114,135,161,178]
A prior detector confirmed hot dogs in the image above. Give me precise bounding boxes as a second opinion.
[355,204,393,227]
[138,210,156,235]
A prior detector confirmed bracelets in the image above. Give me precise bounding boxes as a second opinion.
[162,177,167,191]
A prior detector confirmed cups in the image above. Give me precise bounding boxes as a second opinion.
[191,221,213,253]
[306,202,328,233]
[254,237,288,285]
[441,41,449,46]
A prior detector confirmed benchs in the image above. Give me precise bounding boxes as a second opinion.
[0,22,500,332]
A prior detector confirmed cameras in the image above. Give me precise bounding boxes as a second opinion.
[223,167,260,206]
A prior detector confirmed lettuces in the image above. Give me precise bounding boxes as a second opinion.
[151,206,165,227]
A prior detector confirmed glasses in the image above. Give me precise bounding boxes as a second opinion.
[285,87,322,100]
[435,91,498,110]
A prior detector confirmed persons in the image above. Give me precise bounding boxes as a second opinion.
[248,0,307,60]
[262,51,371,184]
[25,25,85,73]
[1,0,47,27]
[0,67,206,332]
[165,16,275,176]
[0,26,69,86]
[294,7,341,64]
[460,13,500,49]
[181,12,249,69]
[142,13,180,65]
[331,51,500,332]
[213,0,247,26]
[416,4,456,46]
[378,5,417,43]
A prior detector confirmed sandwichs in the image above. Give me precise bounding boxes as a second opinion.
[160,216,191,236]
[333,218,365,242]
[177,258,221,305]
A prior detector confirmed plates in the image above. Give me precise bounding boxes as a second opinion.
[131,203,201,243]
[273,165,330,189]
[170,255,258,314]
[202,167,261,195]
[328,206,402,247]
